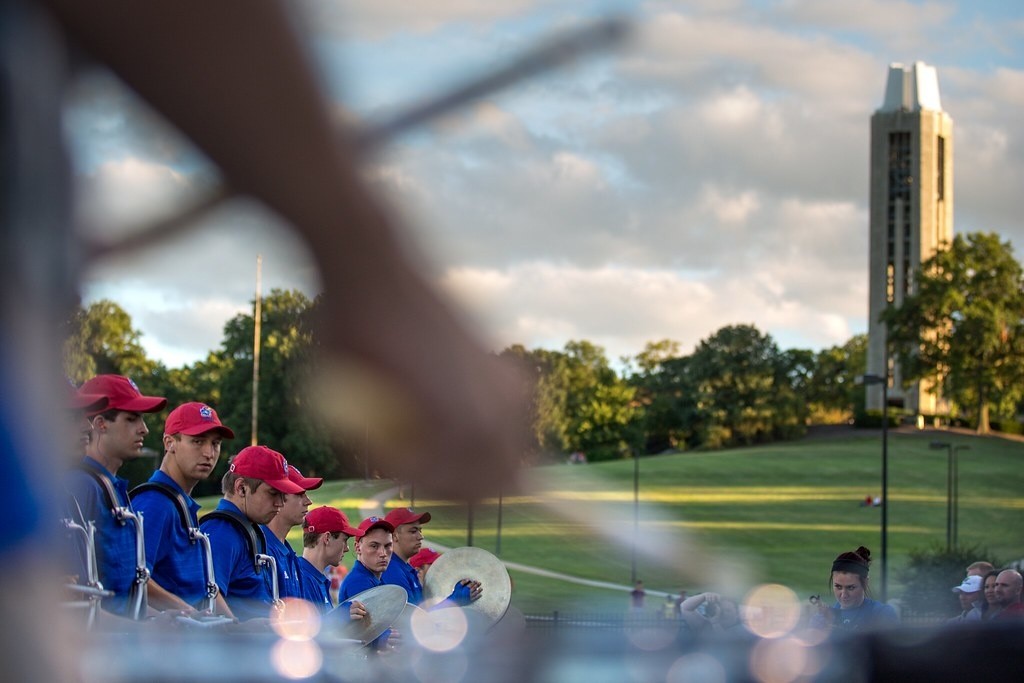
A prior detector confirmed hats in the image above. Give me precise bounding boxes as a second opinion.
[302,507,363,539]
[78,374,169,417]
[385,507,432,529]
[952,574,984,594]
[41,373,109,411]
[355,517,395,542]
[409,547,440,568]
[164,402,236,439]
[284,463,323,491]
[231,445,306,495]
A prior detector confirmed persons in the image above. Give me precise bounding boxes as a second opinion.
[858,494,872,508]
[661,590,687,618]
[47,374,214,636]
[127,403,239,634]
[873,496,881,507]
[408,547,442,588]
[808,545,900,683]
[945,561,1024,625]
[198,446,367,641]
[680,593,759,642]
[629,581,645,610]
[258,465,323,599]
[338,516,395,605]
[0,0,531,683]
[384,507,483,612]
[566,450,585,465]
[297,505,404,656]
[371,467,381,480]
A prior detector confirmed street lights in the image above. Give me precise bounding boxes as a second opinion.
[854,373,889,603]
[927,440,974,554]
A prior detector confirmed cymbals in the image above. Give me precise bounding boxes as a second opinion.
[421,548,512,629]
[360,603,430,665]
[323,584,408,656]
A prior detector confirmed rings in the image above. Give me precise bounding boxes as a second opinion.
[391,644,395,650]
